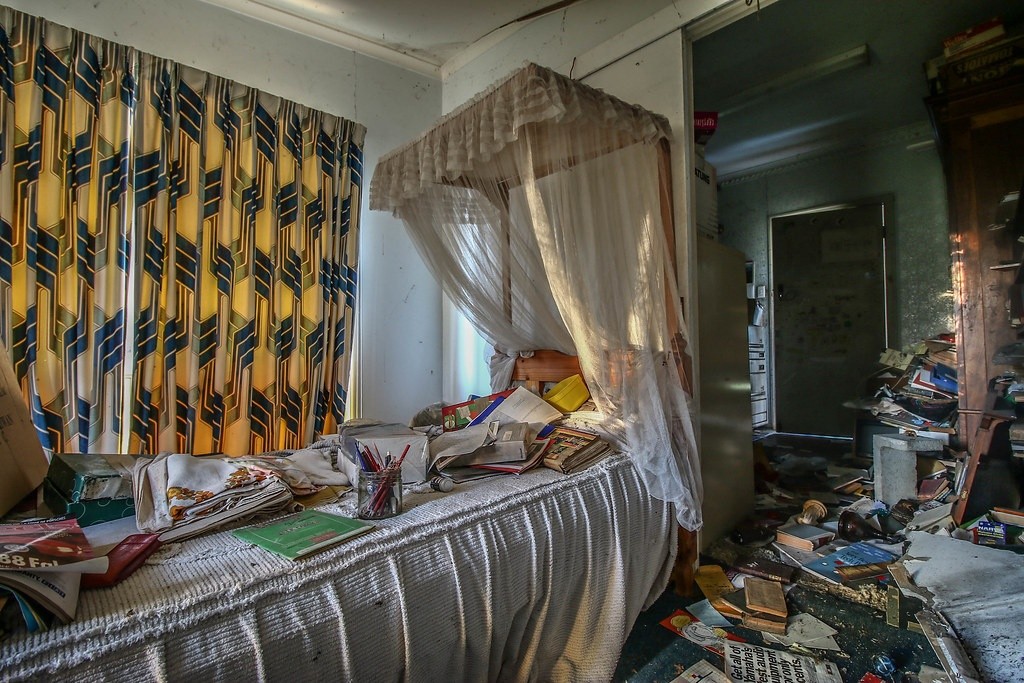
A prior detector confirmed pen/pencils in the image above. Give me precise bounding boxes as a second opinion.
[353,439,412,520]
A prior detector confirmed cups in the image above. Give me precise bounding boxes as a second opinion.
[357,468,403,519]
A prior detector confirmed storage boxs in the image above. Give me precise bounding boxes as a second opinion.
[447,422,530,467]
[542,374,590,412]
[337,422,429,489]
[922,17,1024,95]
[0,66,705,683]
[43,453,158,528]
[0,340,49,518]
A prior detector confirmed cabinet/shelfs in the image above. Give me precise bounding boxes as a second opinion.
[747,326,769,428]
[921,66,1024,449]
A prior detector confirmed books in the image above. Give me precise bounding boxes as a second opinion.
[0,510,110,639]
[677,326,1024,680]
[433,381,623,481]
